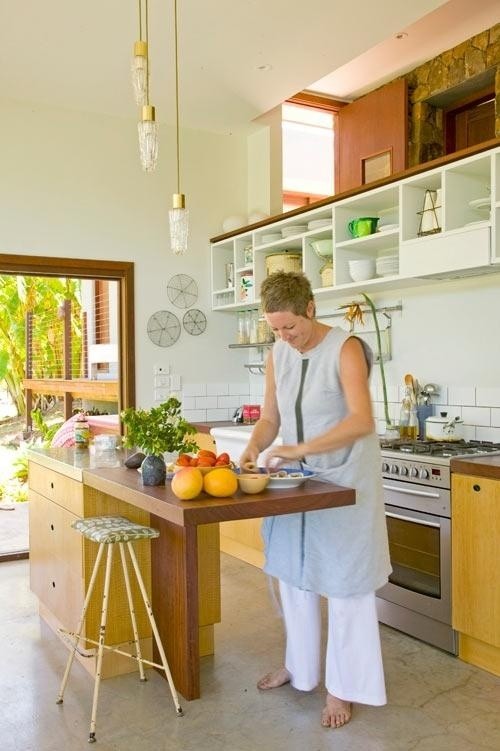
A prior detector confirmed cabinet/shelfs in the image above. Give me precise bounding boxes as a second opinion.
[210,147,500,316]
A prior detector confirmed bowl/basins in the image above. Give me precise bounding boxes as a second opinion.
[238,474,269,495]
[347,260,375,283]
[173,461,232,476]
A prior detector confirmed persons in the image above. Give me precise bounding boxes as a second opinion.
[237,269,396,729]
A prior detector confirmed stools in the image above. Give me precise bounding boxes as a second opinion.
[58,517,184,743]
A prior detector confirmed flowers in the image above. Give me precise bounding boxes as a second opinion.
[334,290,391,424]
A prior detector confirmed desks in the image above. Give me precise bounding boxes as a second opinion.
[26,431,358,705]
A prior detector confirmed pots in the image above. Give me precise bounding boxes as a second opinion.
[424,412,467,443]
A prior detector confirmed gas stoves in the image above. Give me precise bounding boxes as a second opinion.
[379,438,500,490]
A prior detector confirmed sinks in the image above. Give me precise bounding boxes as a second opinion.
[210,425,282,468]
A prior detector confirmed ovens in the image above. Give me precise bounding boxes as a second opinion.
[369,478,453,624]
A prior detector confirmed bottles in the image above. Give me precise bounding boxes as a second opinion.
[94,437,116,452]
[235,307,276,343]
[398,400,419,439]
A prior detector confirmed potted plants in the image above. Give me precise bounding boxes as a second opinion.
[120,400,199,487]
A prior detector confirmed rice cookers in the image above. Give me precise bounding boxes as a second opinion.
[263,249,301,277]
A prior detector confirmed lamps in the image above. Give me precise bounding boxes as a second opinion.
[168,0,189,256]
[130,0,158,176]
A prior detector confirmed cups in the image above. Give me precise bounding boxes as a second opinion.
[225,263,234,287]
[214,292,234,306]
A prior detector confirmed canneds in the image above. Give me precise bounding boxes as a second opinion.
[73,419,90,451]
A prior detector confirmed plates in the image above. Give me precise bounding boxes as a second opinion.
[378,223,399,233]
[137,463,176,480]
[470,198,491,211]
[258,217,332,242]
[465,219,488,226]
[232,467,316,491]
[374,256,399,277]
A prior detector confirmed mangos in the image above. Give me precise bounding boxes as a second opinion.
[178,450,229,467]
